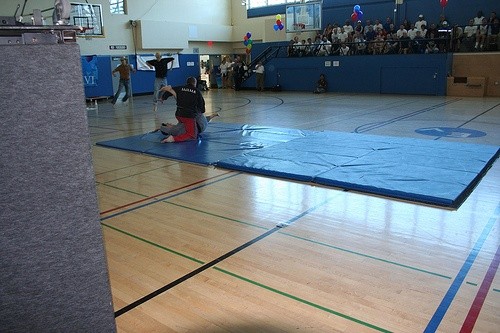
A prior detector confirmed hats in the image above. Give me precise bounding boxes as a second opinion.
[155,52,162,57]
[120,56,128,62]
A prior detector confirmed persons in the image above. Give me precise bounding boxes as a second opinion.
[160,77,205,143]
[255,61,265,91]
[148,85,221,141]
[317,74,327,92]
[221,56,250,91]
[289,11,500,54]
[146,52,175,112]
[111,57,136,108]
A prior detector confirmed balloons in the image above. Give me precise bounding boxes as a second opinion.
[440,0,447,7]
[243,32,253,56]
[351,5,363,21]
[273,14,284,31]
